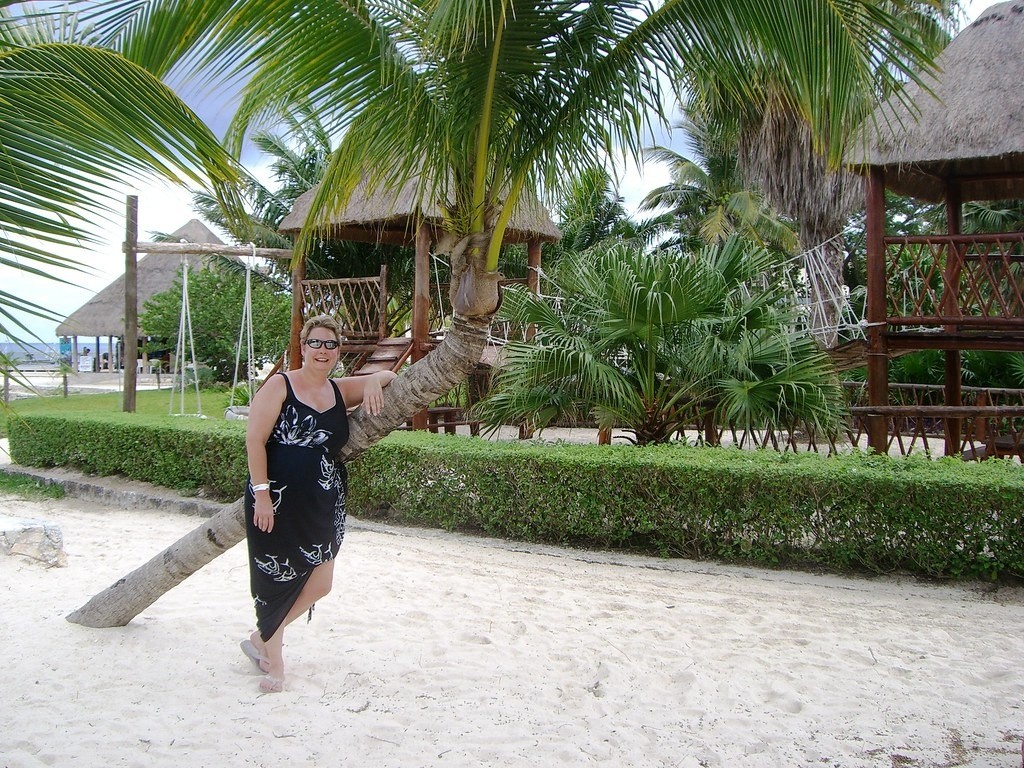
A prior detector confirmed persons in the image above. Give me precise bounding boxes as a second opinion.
[240,315,397,694]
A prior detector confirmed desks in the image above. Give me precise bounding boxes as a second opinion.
[405,407,463,434]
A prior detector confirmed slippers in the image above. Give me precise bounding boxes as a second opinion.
[259,674,286,693]
[240,639,271,675]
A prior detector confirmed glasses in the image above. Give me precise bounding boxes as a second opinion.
[304,338,339,350]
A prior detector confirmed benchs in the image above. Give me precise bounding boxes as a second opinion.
[398,421,485,437]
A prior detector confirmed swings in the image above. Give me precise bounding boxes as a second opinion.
[167,252,208,420]
[224,256,257,421]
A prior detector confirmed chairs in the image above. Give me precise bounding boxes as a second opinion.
[136,359,162,374]
[78,356,93,372]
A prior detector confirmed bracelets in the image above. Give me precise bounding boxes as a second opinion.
[252,484,270,492]
